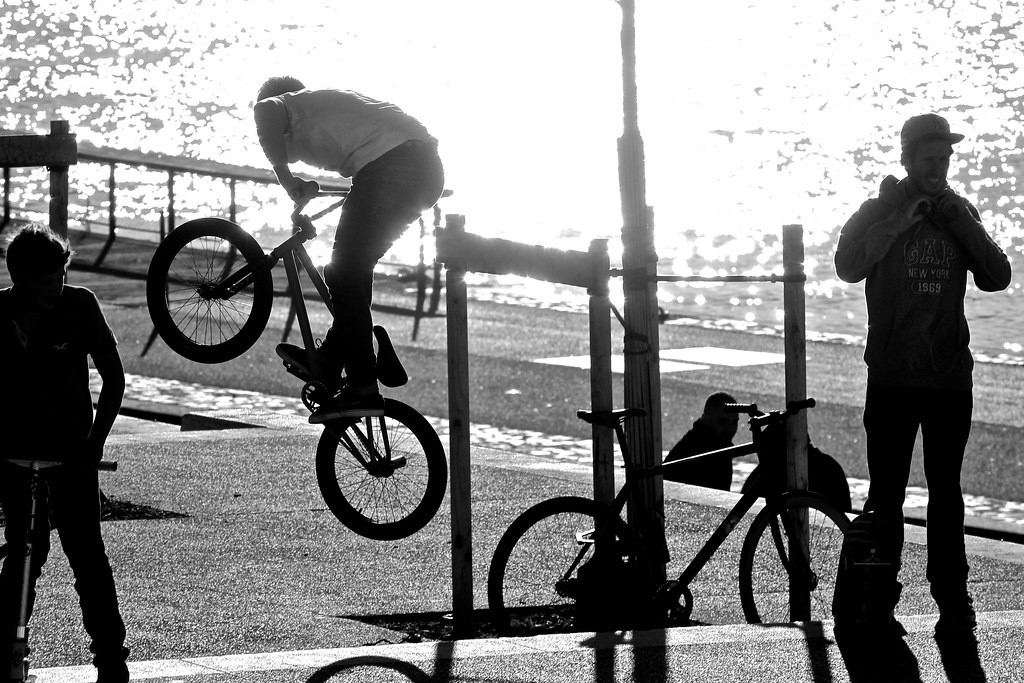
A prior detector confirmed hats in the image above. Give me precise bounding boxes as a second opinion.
[901,113,966,151]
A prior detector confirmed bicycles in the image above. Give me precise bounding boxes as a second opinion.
[147,185,447,540]
[488,399,850,637]
[0,456,117,683]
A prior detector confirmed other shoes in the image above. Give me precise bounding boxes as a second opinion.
[868,614,892,626]
[932,590,976,623]
[95,661,130,682]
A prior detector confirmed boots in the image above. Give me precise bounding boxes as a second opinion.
[275,322,350,388]
[308,353,386,423]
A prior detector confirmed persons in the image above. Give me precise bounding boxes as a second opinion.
[834,114,1012,629]
[254,76,444,423]
[0,225,130,683]
